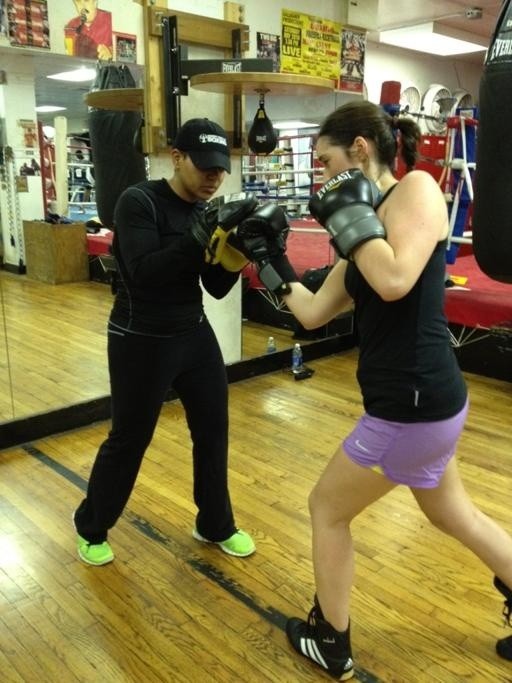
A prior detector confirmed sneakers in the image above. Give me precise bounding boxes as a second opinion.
[191,522,257,558]
[71,508,116,567]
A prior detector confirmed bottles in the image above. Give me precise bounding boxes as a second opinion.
[268,337,275,353]
[292,343,303,374]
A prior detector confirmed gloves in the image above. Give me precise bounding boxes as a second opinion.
[191,194,260,267]
[306,165,388,260]
[234,201,300,295]
[219,202,289,273]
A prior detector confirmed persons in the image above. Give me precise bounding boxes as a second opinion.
[232,99,510,680]
[64,0,114,60]
[75,117,257,566]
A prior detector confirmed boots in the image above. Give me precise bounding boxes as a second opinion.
[286,590,357,681]
[492,573,512,661]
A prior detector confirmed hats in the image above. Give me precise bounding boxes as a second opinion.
[173,116,232,174]
